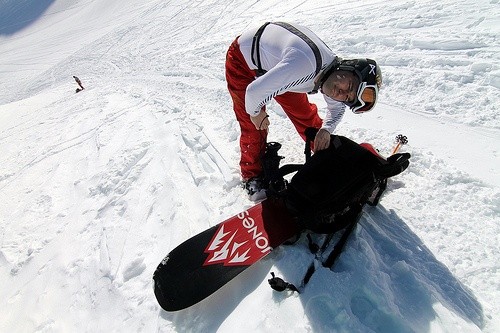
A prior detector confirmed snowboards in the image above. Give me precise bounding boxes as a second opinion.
[152,142,383,312]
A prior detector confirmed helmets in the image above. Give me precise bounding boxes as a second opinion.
[338,58,382,112]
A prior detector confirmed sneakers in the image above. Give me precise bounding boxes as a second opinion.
[243,176,267,201]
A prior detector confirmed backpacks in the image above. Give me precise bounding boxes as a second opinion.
[274,129,410,234]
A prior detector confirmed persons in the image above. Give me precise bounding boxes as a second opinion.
[225,21,382,202]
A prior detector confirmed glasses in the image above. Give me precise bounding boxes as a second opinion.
[352,58,377,114]
